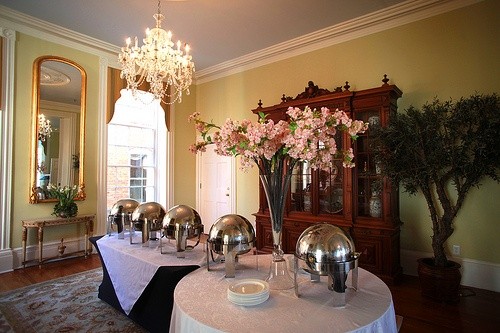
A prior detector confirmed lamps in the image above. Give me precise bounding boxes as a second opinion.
[37,113,53,142]
[117,0,196,104]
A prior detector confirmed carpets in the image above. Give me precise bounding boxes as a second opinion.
[0,267,404,333]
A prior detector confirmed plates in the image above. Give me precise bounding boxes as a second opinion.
[227,278,270,306]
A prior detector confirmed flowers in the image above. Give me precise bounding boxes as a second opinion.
[187,106,369,233]
[49,184,80,215]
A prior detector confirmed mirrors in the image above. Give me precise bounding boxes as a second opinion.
[30,56,87,203]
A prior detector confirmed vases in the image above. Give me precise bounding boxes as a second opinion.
[256,174,296,290]
[58,205,77,219]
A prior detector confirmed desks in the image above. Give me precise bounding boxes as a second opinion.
[21,213,96,270]
[88,231,226,333]
[169,253,399,333]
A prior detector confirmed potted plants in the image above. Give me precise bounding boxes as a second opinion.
[364,93,500,301]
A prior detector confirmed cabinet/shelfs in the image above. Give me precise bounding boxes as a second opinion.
[251,74,404,288]
